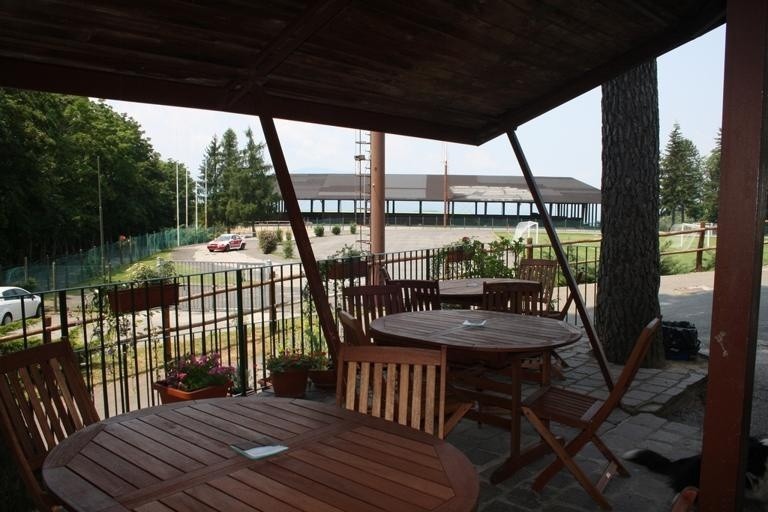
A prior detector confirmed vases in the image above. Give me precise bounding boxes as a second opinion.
[153,380,235,404]
[309,371,336,386]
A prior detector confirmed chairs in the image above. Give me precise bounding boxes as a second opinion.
[1,336,101,511]
[521,314,662,511]
[508,257,557,312]
[539,272,583,367]
[381,267,390,282]
[385,278,441,312]
[482,281,543,317]
[336,308,473,439]
[341,283,404,341]
[336,342,447,440]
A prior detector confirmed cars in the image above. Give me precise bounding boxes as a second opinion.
[0,287,42,324]
[207,234,245,252]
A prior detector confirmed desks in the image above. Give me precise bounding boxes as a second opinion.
[41,396,480,512]
[415,278,538,310]
[370,310,576,485]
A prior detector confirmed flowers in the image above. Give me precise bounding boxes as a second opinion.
[308,351,331,370]
[155,351,235,388]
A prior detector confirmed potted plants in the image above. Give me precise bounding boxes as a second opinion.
[87,264,180,377]
[430,236,475,281]
[302,245,368,351]
[266,347,311,398]
